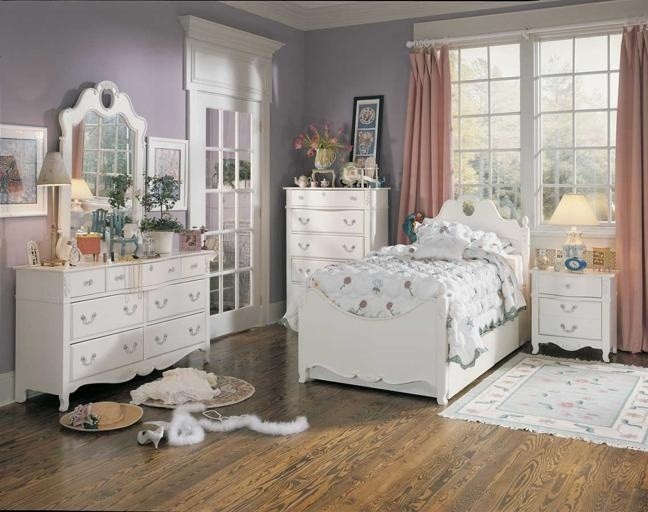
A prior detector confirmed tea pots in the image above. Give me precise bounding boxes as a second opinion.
[293,174,311,188]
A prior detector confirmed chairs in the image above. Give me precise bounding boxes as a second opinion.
[108,208,139,261]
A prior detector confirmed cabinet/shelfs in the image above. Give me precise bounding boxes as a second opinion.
[13,246,217,413]
[284,184,389,323]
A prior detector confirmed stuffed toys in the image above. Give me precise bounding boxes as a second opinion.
[403,209,424,243]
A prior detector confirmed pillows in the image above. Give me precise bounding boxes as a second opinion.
[413,217,510,261]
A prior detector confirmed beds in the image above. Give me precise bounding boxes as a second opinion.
[276,196,531,407]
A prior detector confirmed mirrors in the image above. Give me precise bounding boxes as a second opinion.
[56,79,149,260]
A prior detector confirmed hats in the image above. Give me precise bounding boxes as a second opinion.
[59,401,144,433]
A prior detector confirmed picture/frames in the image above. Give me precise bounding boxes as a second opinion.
[348,93,382,170]
[0,120,49,220]
[178,227,200,247]
[142,132,189,214]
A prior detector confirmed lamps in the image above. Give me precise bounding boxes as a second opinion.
[545,190,601,265]
[68,175,91,229]
[32,148,76,267]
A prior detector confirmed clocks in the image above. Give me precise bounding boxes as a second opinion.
[564,257,586,274]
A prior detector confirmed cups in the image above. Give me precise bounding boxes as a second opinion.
[309,178,329,188]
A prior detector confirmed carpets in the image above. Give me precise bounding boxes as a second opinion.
[435,349,646,453]
[136,371,255,414]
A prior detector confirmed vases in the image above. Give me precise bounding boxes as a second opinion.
[313,147,334,169]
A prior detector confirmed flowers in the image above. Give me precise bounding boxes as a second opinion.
[293,119,355,163]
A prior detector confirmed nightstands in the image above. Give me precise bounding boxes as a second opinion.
[526,262,621,367]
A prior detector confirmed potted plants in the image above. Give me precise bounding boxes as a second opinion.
[130,174,183,256]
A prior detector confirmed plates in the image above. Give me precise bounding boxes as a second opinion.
[340,162,360,185]
